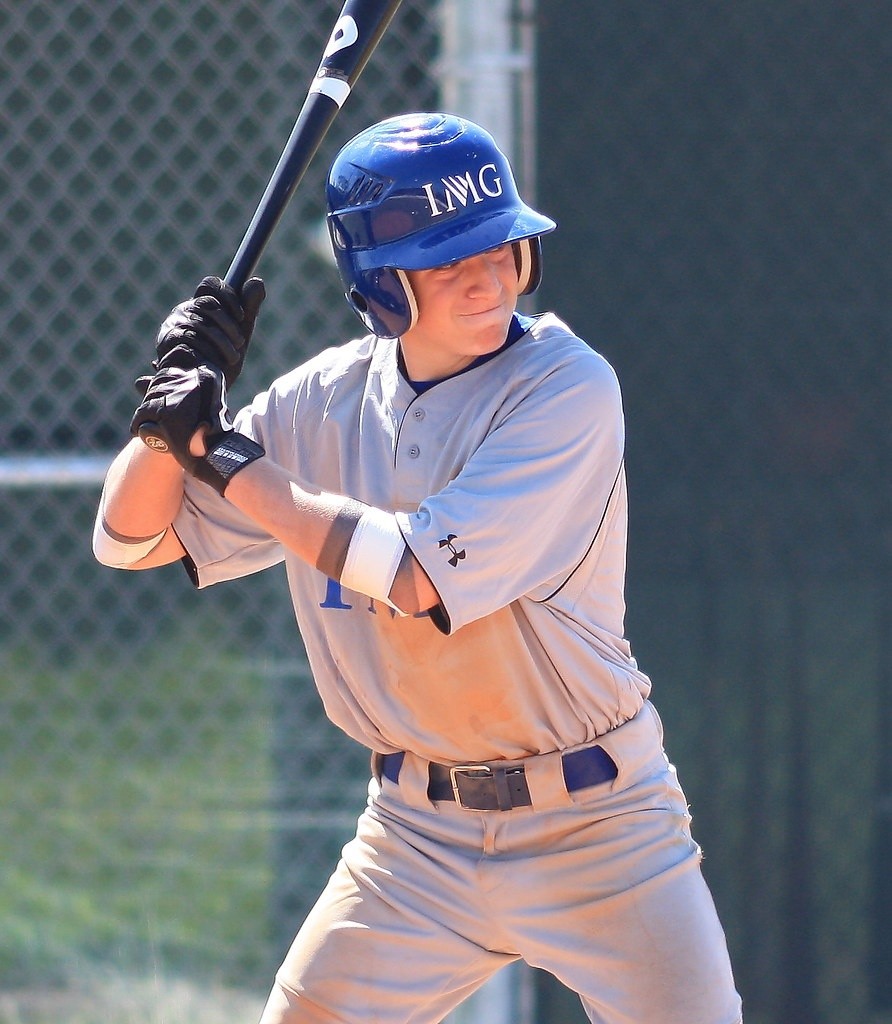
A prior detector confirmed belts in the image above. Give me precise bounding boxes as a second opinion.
[378,745,617,812]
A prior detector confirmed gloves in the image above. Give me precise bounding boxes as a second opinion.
[129,344,267,501]
[156,275,266,391]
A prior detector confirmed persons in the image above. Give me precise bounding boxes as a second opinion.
[92,114,743,1023]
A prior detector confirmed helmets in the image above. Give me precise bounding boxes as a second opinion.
[326,114,558,340]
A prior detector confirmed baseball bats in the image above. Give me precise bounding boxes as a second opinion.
[138,1,402,451]
